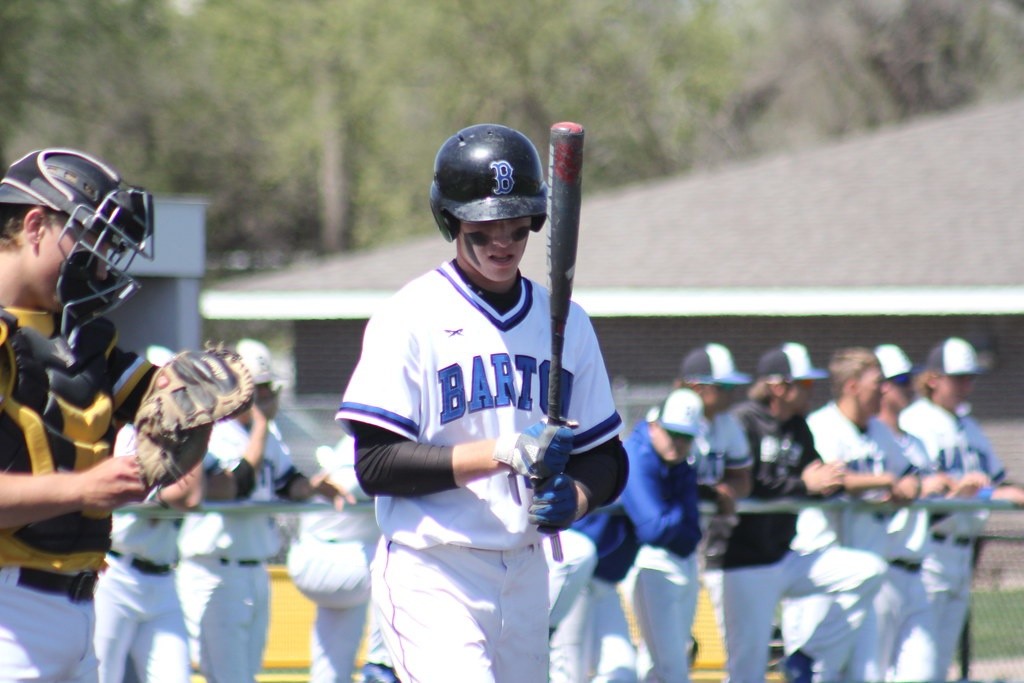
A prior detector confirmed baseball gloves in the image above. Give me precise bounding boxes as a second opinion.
[125,337,255,509]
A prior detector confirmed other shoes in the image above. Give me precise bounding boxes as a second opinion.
[778,654,814,683]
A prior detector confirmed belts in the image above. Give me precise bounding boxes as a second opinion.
[18,567,96,601]
[934,533,970,544]
[220,556,260,567]
[893,558,922,572]
[108,550,171,575]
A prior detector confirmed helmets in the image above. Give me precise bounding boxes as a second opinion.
[430,124,548,242]
[0,148,146,253]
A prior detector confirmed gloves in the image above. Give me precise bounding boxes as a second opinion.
[527,474,578,534]
[492,417,579,479]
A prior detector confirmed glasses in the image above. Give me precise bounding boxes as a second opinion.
[890,376,908,385]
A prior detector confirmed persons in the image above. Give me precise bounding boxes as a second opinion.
[0,143,382,683]
[541,337,1024,683]
[335,123,628,683]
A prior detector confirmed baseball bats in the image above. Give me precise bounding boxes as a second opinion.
[534,121,589,535]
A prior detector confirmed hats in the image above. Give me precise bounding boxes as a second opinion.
[234,337,276,390]
[757,344,829,382]
[659,388,704,435]
[682,343,753,385]
[928,337,989,376]
[874,345,927,378]
